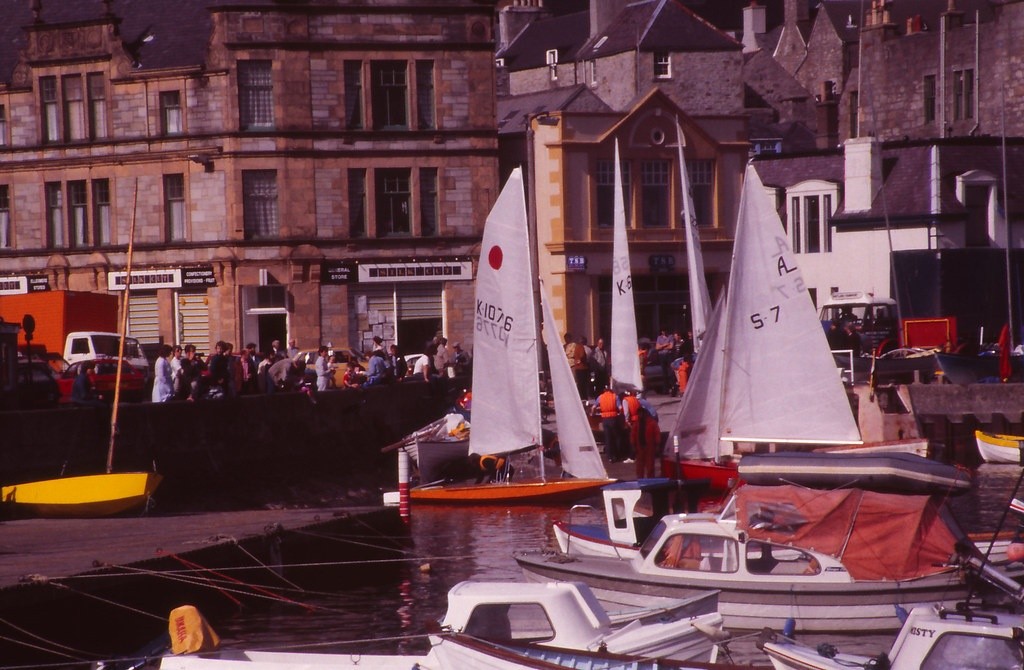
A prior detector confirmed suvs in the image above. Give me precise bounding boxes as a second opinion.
[18,344,70,388]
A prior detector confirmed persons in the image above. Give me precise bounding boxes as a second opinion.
[636,328,694,399]
[71,360,103,405]
[630,406,660,479]
[444,407,464,437]
[1005,516,1024,566]
[474,454,506,485]
[150,329,473,402]
[591,385,658,461]
[822,306,884,370]
[563,333,607,368]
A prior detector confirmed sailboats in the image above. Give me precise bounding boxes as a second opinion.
[661,163,862,495]
[385,165,620,501]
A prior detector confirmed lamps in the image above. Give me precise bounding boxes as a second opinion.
[189,154,209,166]
[131,32,154,52]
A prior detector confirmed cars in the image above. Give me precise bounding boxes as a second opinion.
[639,336,664,392]
[290,345,371,390]
[57,359,145,400]
[396,353,455,378]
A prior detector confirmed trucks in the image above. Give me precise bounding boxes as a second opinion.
[0,291,150,387]
[820,289,900,358]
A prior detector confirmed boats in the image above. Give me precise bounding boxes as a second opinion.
[977,431,1024,464]
[400,416,469,486]
[0,472,164,518]
[934,349,1024,385]
[513,477,1024,632]
[736,448,975,497]
[817,439,930,459]
[158,565,1024,670]
[878,348,936,386]
[834,355,873,383]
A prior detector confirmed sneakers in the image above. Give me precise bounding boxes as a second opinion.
[623,458,635,464]
